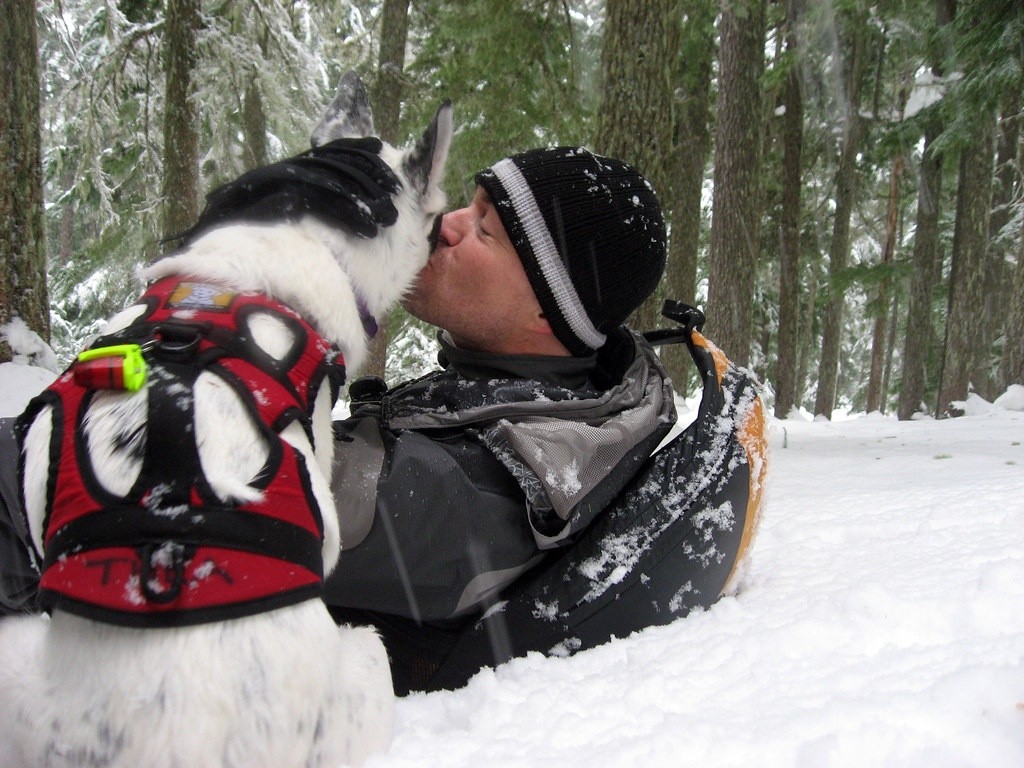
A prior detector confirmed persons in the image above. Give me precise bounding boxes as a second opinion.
[316,149,677,698]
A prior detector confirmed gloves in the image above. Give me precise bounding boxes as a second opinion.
[159,136,406,240]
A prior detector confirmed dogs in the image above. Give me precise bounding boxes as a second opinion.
[18,64,459,768]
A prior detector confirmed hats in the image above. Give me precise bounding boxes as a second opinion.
[474,145,668,358]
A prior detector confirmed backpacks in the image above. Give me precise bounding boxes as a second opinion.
[326,298,770,698]
